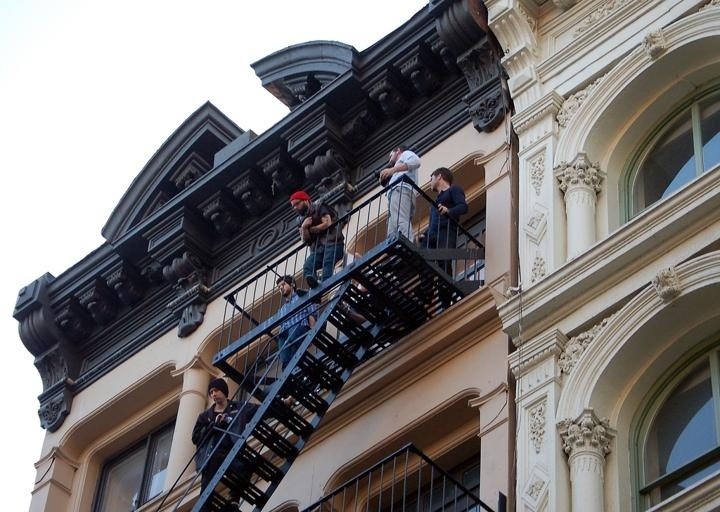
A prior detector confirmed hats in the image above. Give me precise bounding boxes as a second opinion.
[291,191,311,201]
[208,378,229,398]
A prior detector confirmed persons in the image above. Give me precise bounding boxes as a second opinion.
[412,166,470,307]
[378,143,422,270]
[334,251,379,348]
[190,376,296,512]
[271,273,322,393]
[288,190,345,305]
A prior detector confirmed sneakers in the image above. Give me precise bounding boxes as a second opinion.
[296,274,323,305]
